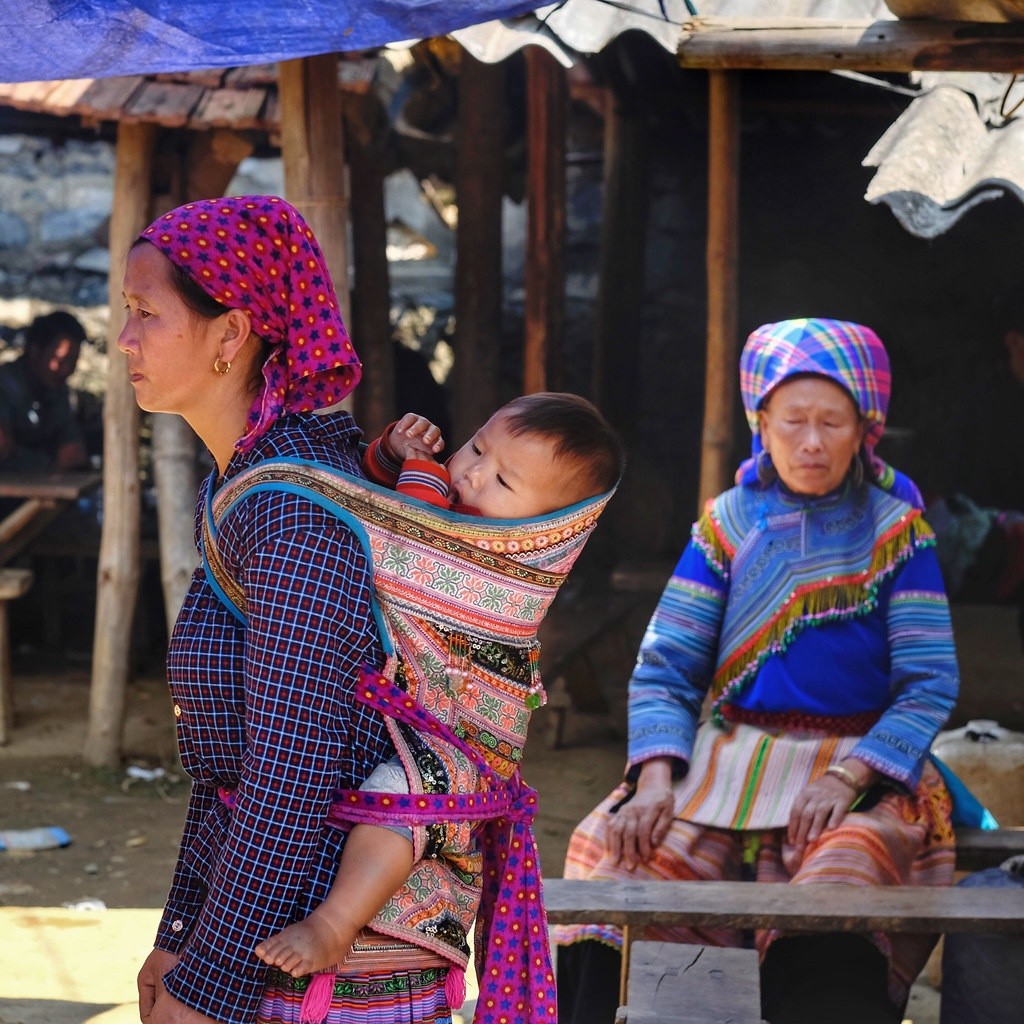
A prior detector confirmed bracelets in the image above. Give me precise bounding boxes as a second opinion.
[824,767,859,792]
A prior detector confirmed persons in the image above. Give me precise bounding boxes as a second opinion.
[116,196,626,1024]
[551,319,959,1024]
[0,311,88,472]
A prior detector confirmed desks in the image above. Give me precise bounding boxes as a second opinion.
[0,464,102,567]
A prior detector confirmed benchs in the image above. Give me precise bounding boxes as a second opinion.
[538,823,1024,1024]
